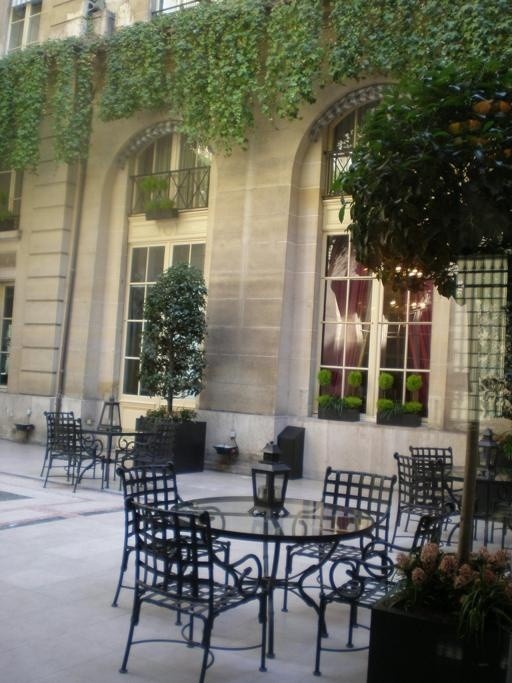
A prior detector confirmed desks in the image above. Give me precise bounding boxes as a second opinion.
[424,468,510,547]
[74,426,154,489]
[169,496,375,660]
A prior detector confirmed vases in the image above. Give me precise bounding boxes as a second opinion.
[367,592,512,682]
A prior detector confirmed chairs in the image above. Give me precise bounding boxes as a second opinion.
[111,461,231,627]
[405,445,459,533]
[118,495,269,683]
[114,415,161,484]
[388,452,459,554]
[118,422,176,491]
[43,418,105,494]
[447,477,511,549]
[310,512,447,676]
[281,465,398,627]
[40,411,96,482]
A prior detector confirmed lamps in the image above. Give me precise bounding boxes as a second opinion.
[248,441,290,519]
[15,409,35,432]
[476,428,501,478]
[97,395,121,432]
[213,431,240,459]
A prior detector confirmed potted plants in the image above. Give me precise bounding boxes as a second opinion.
[317,370,337,420]
[340,371,362,423]
[400,374,423,428]
[134,264,207,475]
[377,373,395,426]
[141,176,181,221]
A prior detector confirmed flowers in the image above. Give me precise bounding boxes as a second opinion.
[392,541,511,630]
